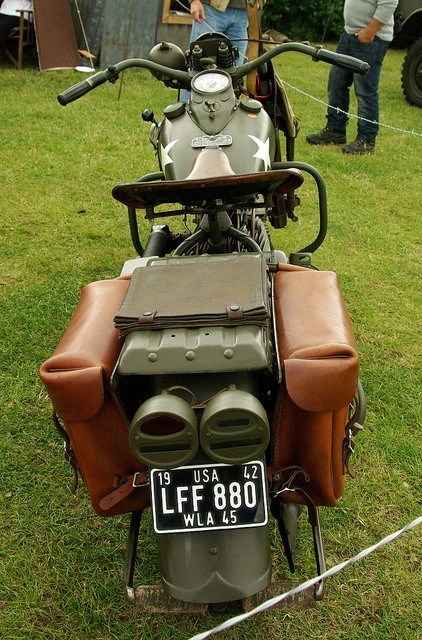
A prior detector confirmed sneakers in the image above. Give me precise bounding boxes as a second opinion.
[341,138,374,155]
[305,128,346,145]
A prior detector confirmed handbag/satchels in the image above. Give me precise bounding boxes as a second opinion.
[260,69,297,139]
[272,263,366,506]
[40,277,149,517]
[113,258,269,338]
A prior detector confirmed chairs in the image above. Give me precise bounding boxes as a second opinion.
[0,8,34,71]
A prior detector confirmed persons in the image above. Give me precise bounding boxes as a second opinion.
[180,0,250,106]
[306,1,399,154]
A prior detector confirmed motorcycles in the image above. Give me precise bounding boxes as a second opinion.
[38,31,370,613]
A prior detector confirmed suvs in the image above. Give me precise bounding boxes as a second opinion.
[393,0,422,107]
